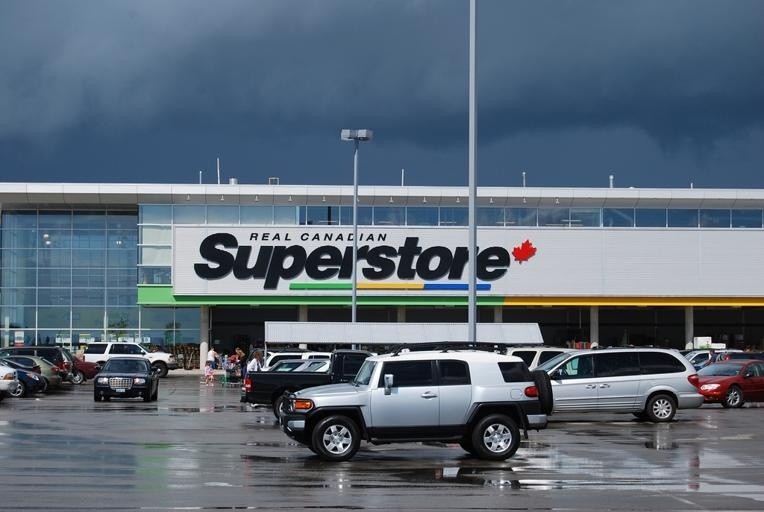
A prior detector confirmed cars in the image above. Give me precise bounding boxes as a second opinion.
[0,355,63,397]
[94,357,159,402]
[685,350,764,408]
[504,347,704,423]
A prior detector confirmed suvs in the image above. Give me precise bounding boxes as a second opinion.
[279,345,554,461]
[81,342,178,378]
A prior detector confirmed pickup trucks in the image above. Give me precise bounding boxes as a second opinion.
[239,349,374,420]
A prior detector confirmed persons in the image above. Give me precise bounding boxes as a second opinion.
[705,349,717,365]
[203,345,261,388]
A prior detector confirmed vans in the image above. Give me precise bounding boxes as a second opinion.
[243,347,337,373]
[0,346,101,384]
